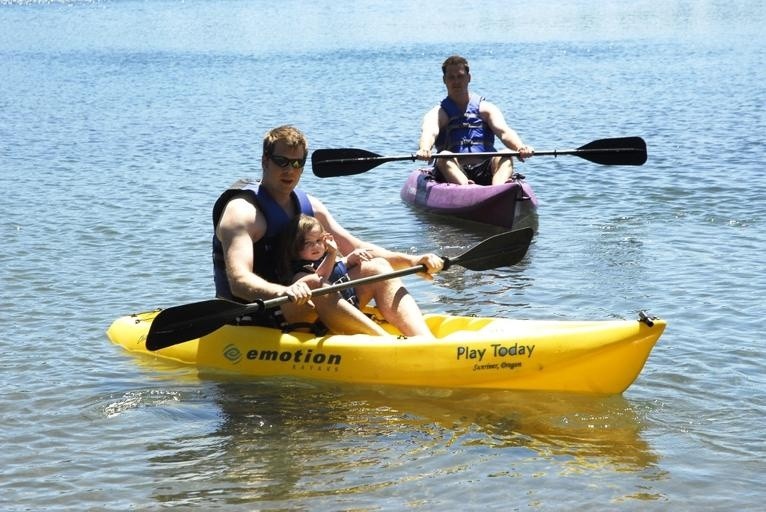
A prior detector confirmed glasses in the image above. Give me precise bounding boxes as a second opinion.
[267,153,304,168]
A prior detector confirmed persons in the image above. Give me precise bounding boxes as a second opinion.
[209,123,444,338]
[410,56,533,186]
[282,215,374,342]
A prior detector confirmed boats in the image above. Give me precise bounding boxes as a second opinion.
[107,309,667,395]
[401,167,540,232]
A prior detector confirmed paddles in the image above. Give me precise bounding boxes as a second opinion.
[312,136,647,178]
[146,226,533,352]
